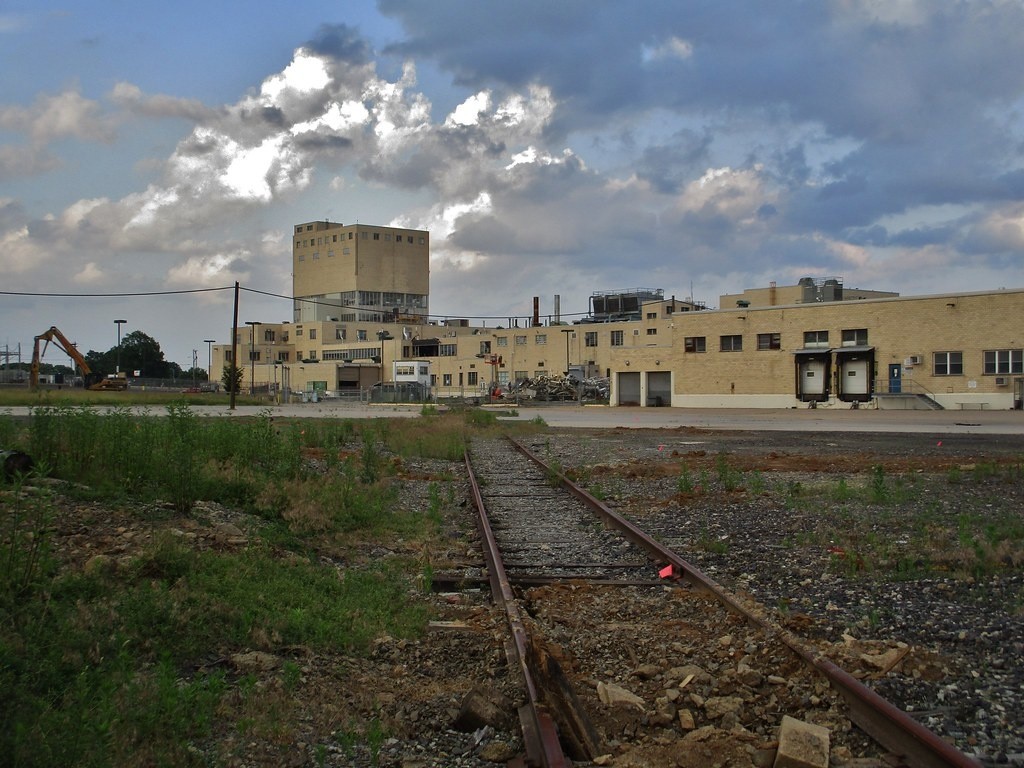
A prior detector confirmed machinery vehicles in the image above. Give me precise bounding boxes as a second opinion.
[29,326,128,392]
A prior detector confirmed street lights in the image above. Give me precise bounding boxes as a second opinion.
[114,319,127,370]
[245,322,262,395]
[561,329,575,372]
[204,340,216,382]
